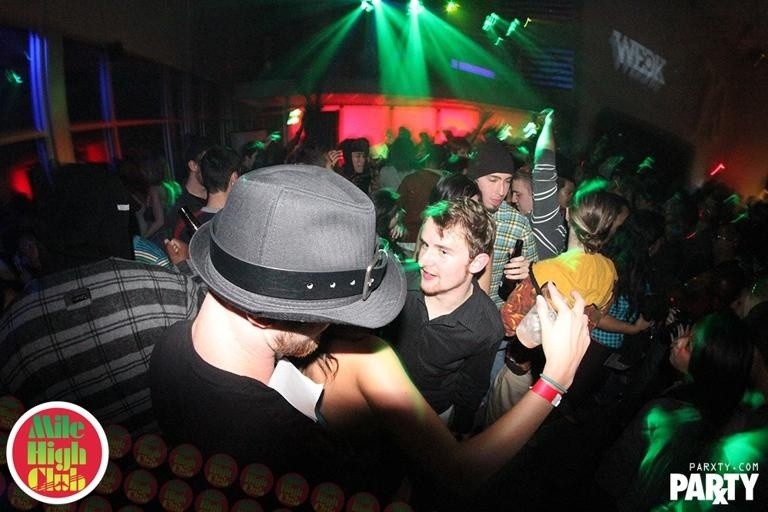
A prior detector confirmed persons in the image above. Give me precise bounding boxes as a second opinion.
[1,107,768,512]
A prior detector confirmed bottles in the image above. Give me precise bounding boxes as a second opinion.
[178,205,202,237]
[498,239,524,301]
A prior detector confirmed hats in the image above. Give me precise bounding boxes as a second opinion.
[189,164,408,329]
[467,136,515,183]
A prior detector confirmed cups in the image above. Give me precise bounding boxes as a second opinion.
[515,285,574,349]
